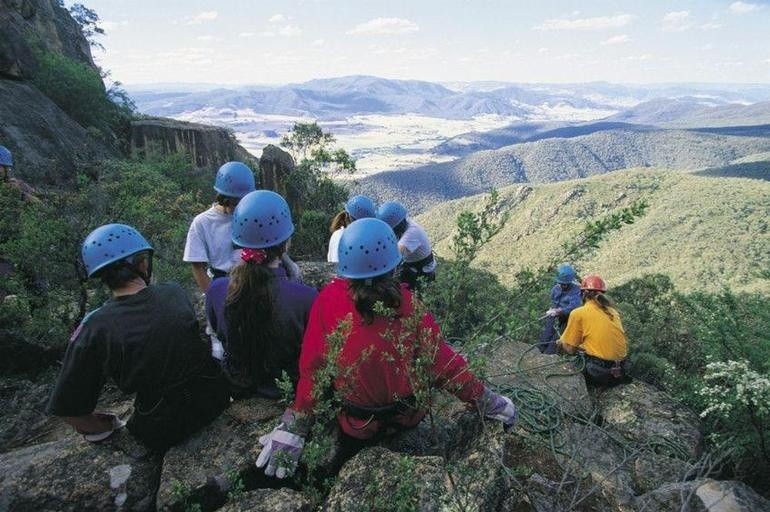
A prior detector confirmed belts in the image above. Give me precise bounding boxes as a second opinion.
[406,253,433,267]
[331,397,415,419]
[588,356,624,369]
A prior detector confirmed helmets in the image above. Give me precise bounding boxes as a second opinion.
[556,266,606,292]
[214,162,295,249]
[82,223,152,278]
[336,195,407,280]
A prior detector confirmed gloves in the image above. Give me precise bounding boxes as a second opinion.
[477,387,514,426]
[256,409,306,478]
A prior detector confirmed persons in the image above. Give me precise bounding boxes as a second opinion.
[0,145,47,209]
[327,195,376,264]
[540,265,585,355]
[182,161,301,360]
[377,201,438,290]
[45,224,231,511]
[256,216,517,479]
[557,276,630,386]
[204,187,323,400]
